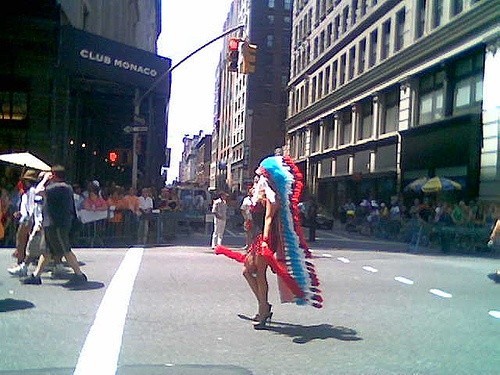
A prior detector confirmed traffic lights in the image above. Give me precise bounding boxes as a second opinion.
[109,147,131,166]
[227,38,239,71]
[241,42,256,74]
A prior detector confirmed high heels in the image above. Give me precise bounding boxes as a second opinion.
[254,305,273,329]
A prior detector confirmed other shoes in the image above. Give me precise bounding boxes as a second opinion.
[19,273,42,285]
[7,262,27,276]
[63,273,87,287]
[48,264,65,276]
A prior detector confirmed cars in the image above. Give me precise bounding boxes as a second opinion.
[297,203,334,227]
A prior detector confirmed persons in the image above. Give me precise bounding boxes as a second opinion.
[183,182,253,249]
[242,176,280,329]
[23,166,86,285]
[339,198,500,251]
[308,197,318,242]
[0,168,179,278]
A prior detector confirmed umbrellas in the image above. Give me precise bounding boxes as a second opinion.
[421,176,461,200]
[403,176,431,196]
[0,151,52,171]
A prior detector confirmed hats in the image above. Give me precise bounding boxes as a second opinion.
[218,191,229,196]
[21,170,37,181]
